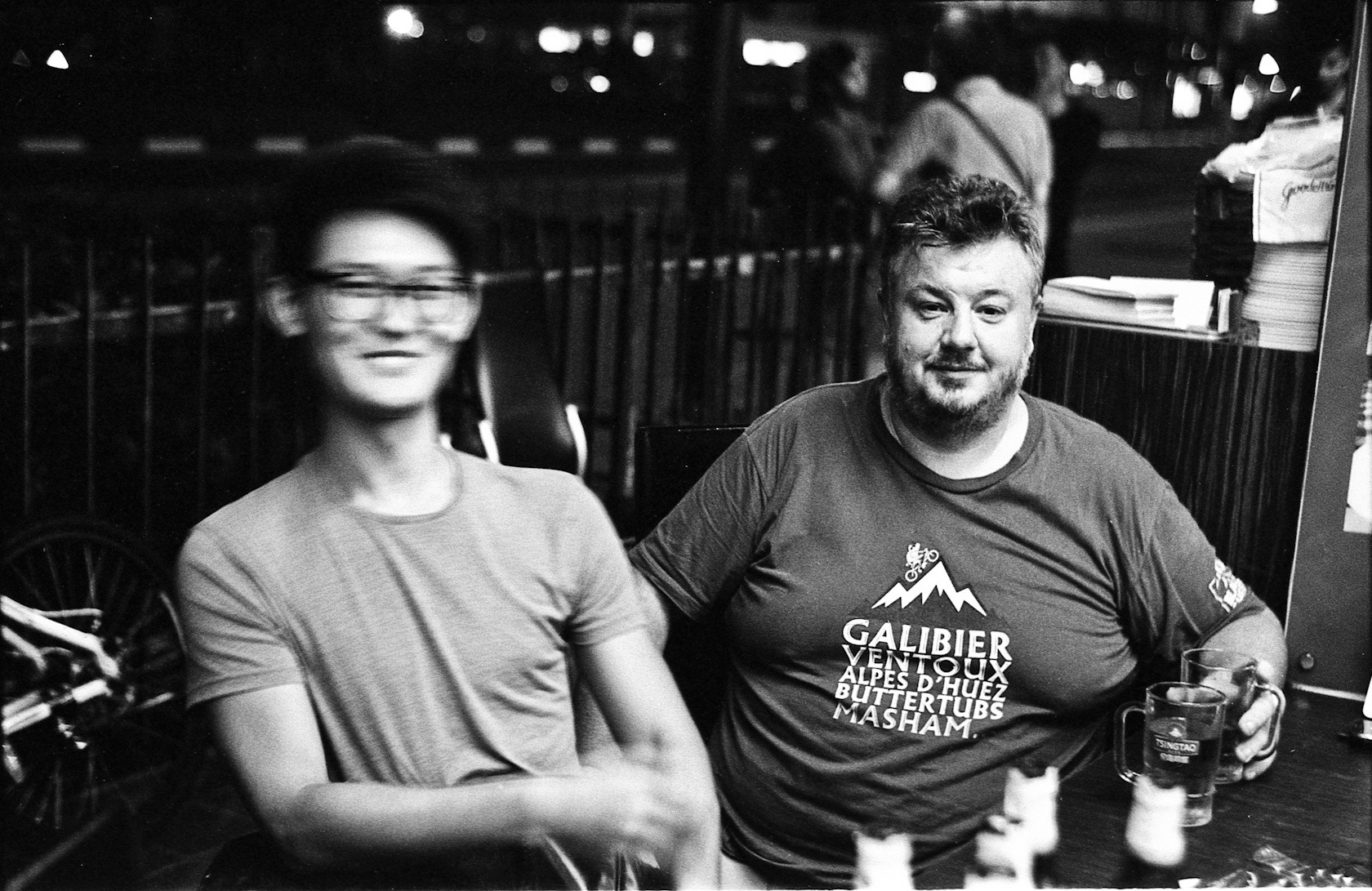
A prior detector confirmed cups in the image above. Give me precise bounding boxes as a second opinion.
[1113,681,1227,828]
[1179,647,1286,786]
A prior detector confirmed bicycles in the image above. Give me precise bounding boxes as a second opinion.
[0,522,193,891]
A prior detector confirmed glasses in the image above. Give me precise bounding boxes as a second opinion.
[292,264,472,326]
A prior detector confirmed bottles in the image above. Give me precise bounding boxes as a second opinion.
[850,817,918,891]
[963,813,1038,891]
[998,758,1064,890]
[1115,770,1190,888]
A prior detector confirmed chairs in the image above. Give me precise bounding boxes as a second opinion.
[159,406,772,891]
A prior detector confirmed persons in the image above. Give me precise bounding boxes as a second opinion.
[1189,28,1354,332]
[180,140,720,891]
[780,33,1101,396]
[574,175,1288,891]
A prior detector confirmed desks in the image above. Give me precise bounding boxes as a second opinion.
[917,686,1372,889]
[1018,311,1261,588]
